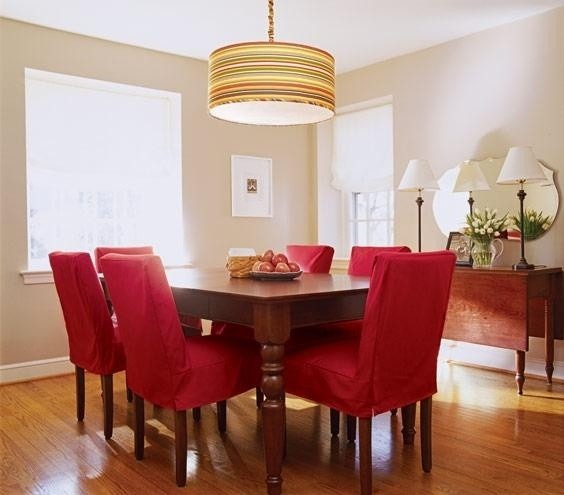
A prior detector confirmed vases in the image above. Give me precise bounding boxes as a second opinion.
[470,238,504,270]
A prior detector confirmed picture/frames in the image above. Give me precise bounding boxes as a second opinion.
[445,231,474,266]
[231,154,273,216]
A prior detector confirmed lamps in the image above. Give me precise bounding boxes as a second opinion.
[453,163,491,218]
[497,146,548,269]
[540,165,555,188]
[398,159,441,253]
[209,1,335,125]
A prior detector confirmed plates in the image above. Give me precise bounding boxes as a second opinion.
[250,269,303,280]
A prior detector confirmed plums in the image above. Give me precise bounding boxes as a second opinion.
[274,261,291,273]
[271,254,289,264]
[259,261,273,272]
[253,260,262,272]
[261,249,274,262]
[288,261,300,272]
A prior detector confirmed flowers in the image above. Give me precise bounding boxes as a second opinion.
[463,207,511,237]
[509,208,554,239]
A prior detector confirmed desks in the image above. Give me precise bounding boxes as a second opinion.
[97,267,417,495]
[441,266,564,394]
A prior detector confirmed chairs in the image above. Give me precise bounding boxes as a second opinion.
[49,252,202,439]
[283,250,457,494]
[98,253,263,487]
[95,246,202,403]
[301,246,412,444]
[212,244,334,406]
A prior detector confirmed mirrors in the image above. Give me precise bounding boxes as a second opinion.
[432,156,559,241]
